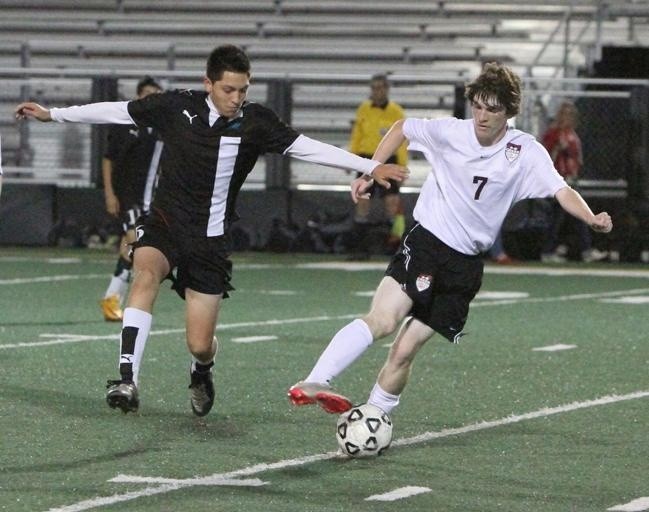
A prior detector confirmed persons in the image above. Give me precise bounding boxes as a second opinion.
[344,75,407,261]
[12,43,411,418]
[286,59,614,454]
[101,79,164,322]
[537,99,609,266]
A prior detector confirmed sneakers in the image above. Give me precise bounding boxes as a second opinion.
[283,379,354,416]
[98,293,125,323]
[104,378,141,416]
[189,361,215,418]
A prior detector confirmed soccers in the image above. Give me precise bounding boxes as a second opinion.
[335,402,393,459]
